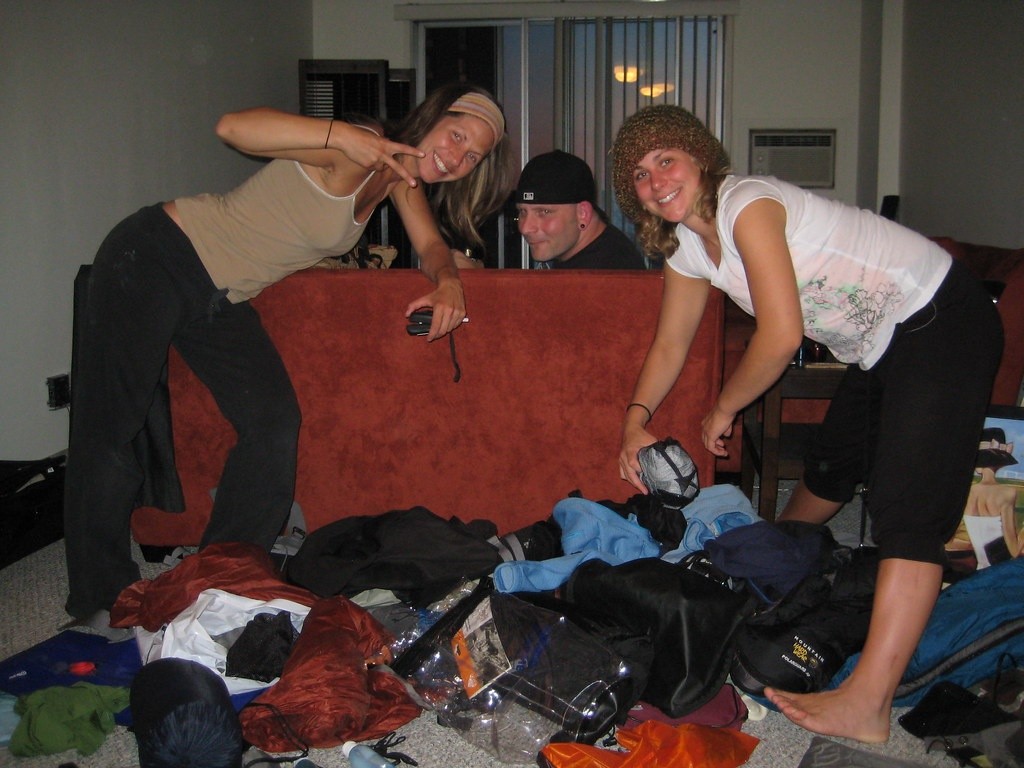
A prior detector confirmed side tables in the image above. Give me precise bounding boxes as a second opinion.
[741,369,873,521]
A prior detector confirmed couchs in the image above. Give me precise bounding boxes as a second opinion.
[931,237,1024,405]
[133,266,731,565]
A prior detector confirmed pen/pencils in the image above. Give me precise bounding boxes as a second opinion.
[462,318,469,322]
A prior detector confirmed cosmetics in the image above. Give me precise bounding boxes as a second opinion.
[342,741,396,768]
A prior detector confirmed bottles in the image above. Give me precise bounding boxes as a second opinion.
[341,740,393,768]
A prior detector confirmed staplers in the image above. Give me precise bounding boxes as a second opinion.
[406,310,434,336]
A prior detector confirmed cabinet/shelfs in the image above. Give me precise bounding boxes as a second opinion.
[296,60,417,269]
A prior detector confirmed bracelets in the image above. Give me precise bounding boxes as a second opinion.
[325,120,332,148]
[626,404,651,422]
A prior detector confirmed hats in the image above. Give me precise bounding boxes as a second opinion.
[515,149,596,205]
[613,104,728,224]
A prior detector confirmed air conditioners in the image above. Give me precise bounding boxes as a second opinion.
[749,131,837,188]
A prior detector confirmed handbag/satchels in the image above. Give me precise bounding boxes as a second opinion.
[1,419,1024,768]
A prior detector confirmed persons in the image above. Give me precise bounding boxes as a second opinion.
[63,74,518,622]
[614,105,1004,743]
[452,149,646,269]
